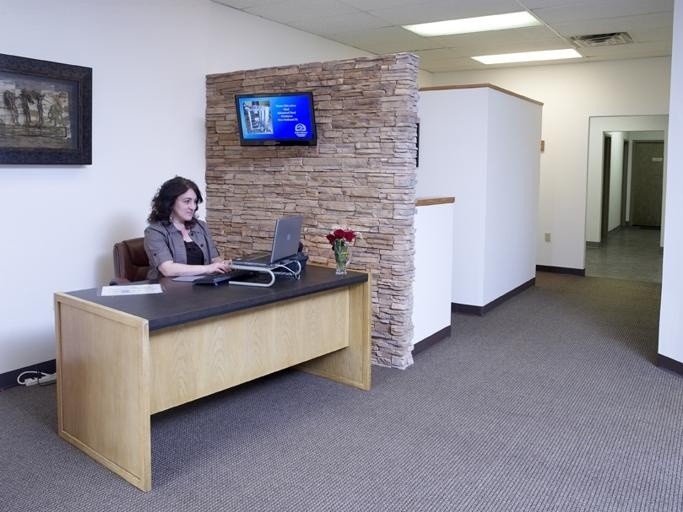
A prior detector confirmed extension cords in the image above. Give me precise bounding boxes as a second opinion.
[25,373,56,387]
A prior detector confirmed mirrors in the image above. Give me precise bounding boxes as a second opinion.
[585,114,669,283]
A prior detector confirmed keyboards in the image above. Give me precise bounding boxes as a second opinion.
[192,270,250,284]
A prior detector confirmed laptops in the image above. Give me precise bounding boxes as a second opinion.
[232,216,304,267]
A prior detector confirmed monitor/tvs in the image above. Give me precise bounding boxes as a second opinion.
[234,92,318,146]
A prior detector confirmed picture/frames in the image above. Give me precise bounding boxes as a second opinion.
[0,54,96,169]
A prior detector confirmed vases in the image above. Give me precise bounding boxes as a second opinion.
[335,248,352,276]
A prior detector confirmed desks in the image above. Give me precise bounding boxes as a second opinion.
[52,256,374,493]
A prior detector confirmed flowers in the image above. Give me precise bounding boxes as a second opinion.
[325,227,356,247]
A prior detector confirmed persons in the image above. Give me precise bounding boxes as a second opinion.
[141,177,233,281]
